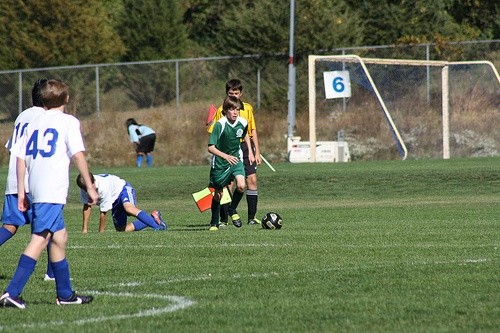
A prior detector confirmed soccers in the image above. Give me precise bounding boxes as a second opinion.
[260,211,284,231]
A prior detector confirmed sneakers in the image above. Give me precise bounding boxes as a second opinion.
[0,293,26,310]
[150,210,167,231]
[55,290,93,305]
[220,220,228,227]
[210,226,218,231]
[227,205,242,227]
[248,218,261,225]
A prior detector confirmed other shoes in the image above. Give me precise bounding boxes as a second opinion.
[44,274,72,282]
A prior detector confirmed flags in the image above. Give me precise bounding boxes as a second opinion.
[206,106,218,125]
[191,185,232,213]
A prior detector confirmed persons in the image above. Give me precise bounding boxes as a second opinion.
[207,78,261,226]
[0,80,98,310]
[208,95,255,231]
[126,117,157,169]
[78,173,167,234]
